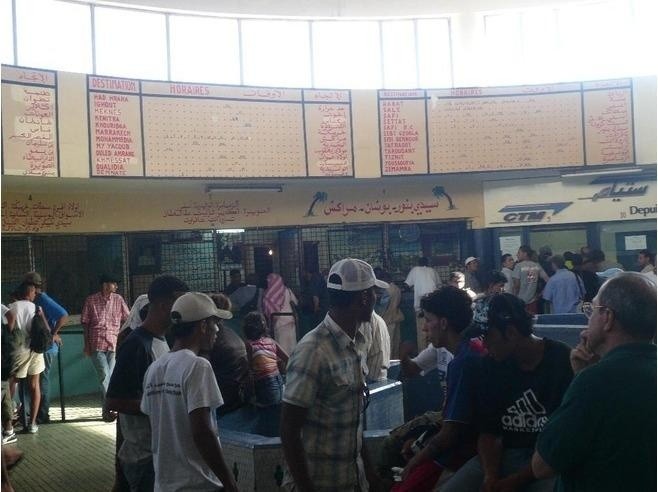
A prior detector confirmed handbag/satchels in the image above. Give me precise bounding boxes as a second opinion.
[31,304,52,353]
[241,297,257,315]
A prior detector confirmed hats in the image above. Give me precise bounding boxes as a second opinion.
[326,257,391,291]
[171,291,232,322]
[462,293,521,337]
[464,258,480,265]
[26,271,42,284]
[596,267,624,279]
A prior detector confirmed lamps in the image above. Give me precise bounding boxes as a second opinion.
[562,166,643,178]
[207,186,283,194]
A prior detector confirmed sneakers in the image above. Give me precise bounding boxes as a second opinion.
[102,411,117,420]
[13,406,48,433]
[2,433,17,445]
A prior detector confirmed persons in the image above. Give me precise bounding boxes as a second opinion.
[9,282,54,434]
[20,272,69,425]
[2,304,19,444]
[101,244,657,492]
[81,274,131,421]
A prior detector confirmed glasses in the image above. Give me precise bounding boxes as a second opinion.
[362,383,370,413]
[583,301,608,318]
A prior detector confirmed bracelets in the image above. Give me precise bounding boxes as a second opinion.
[51,330,58,337]
